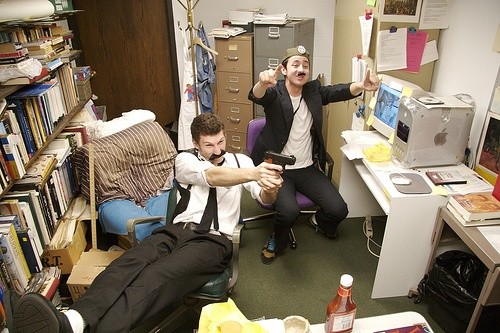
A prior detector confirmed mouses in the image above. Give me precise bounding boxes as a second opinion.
[391,176,411,185]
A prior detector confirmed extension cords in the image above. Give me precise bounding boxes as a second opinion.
[365,215,373,237]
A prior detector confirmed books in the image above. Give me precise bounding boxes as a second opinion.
[207,27,247,38]
[254,13,290,25]
[0,83,65,195]
[446,192,500,227]
[426,170,467,186]
[47,219,75,250]
[53,64,80,115]
[43,126,89,216]
[0,23,82,85]
[0,190,55,324]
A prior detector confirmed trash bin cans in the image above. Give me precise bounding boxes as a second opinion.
[426,249,500,333]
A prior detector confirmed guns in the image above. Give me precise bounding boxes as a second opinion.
[262,150,297,176]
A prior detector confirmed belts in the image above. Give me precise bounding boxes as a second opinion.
[178,221,199,231]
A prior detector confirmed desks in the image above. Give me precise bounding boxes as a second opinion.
[310,310,433,333]
[338,129,494,299]
[408,205,500,333]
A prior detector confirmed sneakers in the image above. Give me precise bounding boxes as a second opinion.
[305,217,338,239]
[262,233,281,264]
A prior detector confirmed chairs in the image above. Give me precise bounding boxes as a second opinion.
[242,117,335,250]
[126,186,246,333]
[74,117,180,250]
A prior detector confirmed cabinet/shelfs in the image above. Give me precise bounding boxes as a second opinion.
[214,32,255,154]
[325,0,440,185]
[253,16,315,118]
[0,12,93,294]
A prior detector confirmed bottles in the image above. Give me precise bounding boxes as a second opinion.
[324,273,357,333]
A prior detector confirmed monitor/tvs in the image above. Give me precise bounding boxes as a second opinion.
[371,74,426,139]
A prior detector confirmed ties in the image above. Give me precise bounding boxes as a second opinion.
[193,187,219,235]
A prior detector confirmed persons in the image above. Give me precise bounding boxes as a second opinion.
[248,45,383,264]
[12,112,283,333]
[452,194,489,203]
[461,202,500,212]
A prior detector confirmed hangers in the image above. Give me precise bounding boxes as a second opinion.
[188,29,219,56]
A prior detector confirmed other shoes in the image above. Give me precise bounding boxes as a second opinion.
[12,292,74,333]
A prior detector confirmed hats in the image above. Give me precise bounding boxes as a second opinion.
[280,46,310,69]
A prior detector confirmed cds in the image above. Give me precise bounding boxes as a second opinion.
[418,97,440,103]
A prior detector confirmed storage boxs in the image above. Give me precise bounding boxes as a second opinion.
[43,222,88,276]
[65,250,125,303]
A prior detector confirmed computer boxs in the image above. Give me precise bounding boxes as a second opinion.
[390,92,475,168]
